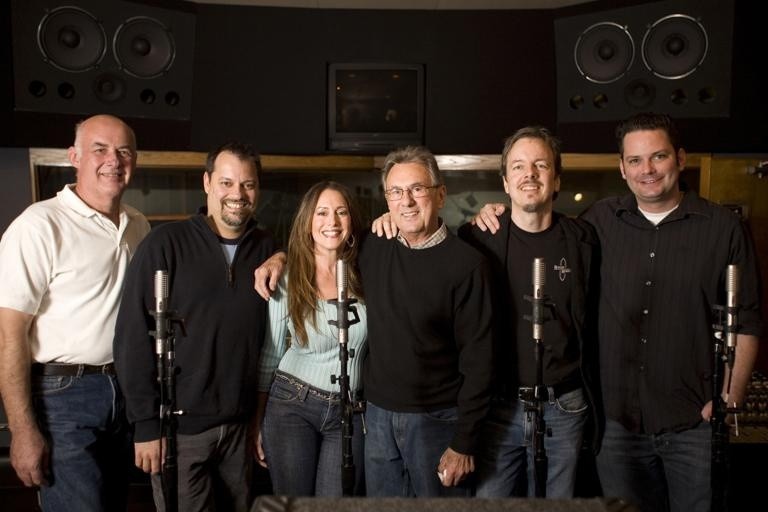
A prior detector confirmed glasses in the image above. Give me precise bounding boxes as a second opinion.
[385,182,443,202]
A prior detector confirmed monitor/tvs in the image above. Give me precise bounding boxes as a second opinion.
[328,62,424,152]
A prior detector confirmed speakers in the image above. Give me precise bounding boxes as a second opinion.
[553,1,735,124]
[11,0,199,126]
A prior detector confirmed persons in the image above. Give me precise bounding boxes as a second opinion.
[253,176,370,497]
[114,135,275,511]
[1,114,154,510]
[372,122,601,500]
[252,143,499,497]
[469,110,761,509]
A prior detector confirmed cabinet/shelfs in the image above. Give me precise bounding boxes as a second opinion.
[26,150,767,278]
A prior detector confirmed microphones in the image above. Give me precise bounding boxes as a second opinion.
[153,269,170,359]
[725,263,739,350]
[335,260,351,346]
[532,258,547,345]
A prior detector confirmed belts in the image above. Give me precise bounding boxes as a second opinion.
[29,359,116,379]
[487,379,585,405]
